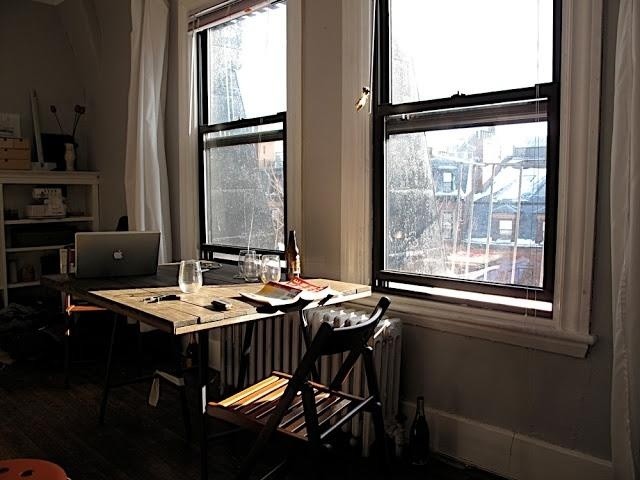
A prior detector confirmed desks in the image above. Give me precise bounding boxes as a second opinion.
[41,258,372,478]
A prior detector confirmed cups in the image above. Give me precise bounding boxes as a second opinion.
[177,259,204,294]
[238,249,282,284]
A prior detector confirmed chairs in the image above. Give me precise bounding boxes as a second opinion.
[202,294,392,478]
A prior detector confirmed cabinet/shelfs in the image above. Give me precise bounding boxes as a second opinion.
[0,167,102,308]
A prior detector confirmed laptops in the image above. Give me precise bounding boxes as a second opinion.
[74,231,161,279]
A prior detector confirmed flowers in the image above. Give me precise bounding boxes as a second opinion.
[50,104,86,143]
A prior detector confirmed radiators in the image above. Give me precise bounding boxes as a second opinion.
[218,306,403,463]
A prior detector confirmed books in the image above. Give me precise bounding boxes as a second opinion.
[68,248,76,274]
[59,248,68,274]
[238,275,332,309]
[25,188,66,220]
[1,111,23,138]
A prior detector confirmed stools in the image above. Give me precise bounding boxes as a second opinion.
[1,459,71,480]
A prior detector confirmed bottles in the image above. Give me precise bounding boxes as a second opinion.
[286,230,301,281]
[185,332,199,368]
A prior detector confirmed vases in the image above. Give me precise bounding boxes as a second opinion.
[64,142,76,171]
